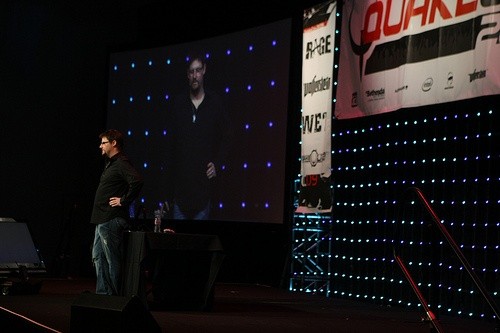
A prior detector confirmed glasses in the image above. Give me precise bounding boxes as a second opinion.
[100,140,113,144]
[186,65,204,77]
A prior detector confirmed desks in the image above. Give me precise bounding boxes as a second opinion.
[119,231,216,310]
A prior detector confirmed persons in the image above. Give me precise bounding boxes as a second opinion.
[159,54,241,209]
[92,130,142,296]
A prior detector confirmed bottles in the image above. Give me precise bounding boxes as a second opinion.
[153,210,162,232]
